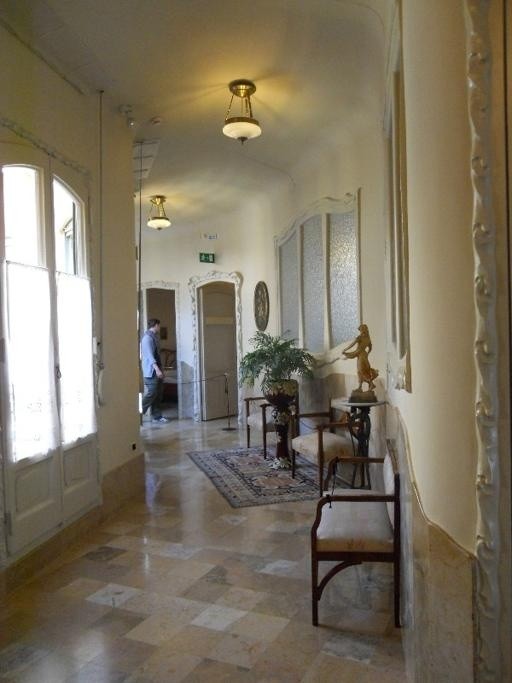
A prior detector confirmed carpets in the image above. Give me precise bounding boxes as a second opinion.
[185,440,355,509]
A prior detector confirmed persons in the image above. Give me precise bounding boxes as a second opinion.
[139,316,169,423]
[343,322,377,391]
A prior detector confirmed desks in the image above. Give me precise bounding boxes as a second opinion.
[340,398,391,489]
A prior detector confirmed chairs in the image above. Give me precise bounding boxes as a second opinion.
[309,440,406,631]
[243,391,304,462]
[287,396,365,492]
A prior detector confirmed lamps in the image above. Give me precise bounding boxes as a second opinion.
[145,196,173,231]
[222,77,263,144]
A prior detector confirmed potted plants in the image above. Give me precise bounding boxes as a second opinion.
[238,329,315,474]
